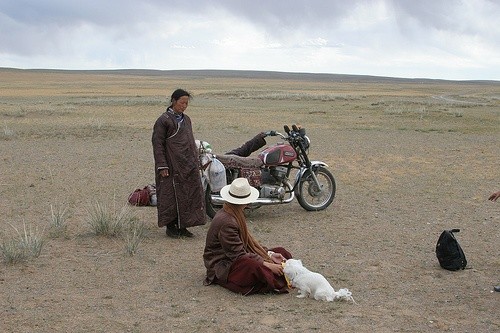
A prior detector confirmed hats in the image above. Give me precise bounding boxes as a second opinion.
[220,179,259,204]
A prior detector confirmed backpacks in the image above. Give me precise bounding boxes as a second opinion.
[436,228,468,271]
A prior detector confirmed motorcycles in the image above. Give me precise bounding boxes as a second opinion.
[205,124,336,220]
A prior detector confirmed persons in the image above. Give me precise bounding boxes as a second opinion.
[203,178,293,295]
[151,88,207,239]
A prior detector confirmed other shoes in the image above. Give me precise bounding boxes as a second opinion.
[166,227,185,238]
[179,227,195,238]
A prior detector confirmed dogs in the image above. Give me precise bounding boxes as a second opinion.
[282,258,358,306]
